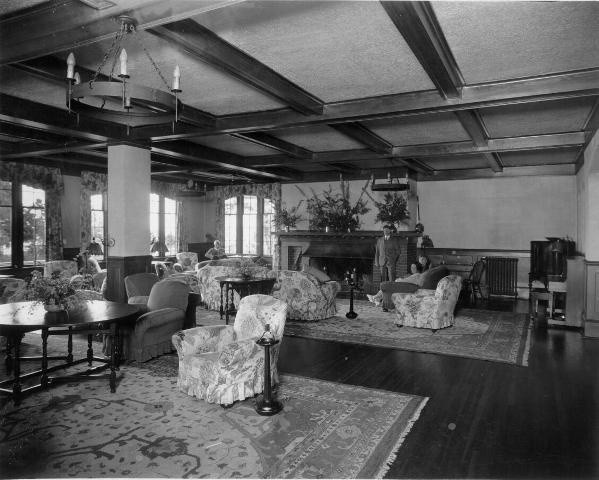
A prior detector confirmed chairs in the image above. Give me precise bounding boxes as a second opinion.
[155,263,197,292]
[0,278,25,304]
[87,258,106,283]
[102,273,189,363]
[462,260,484,304]
[392,275,462,332]
[125,273,202,329]
[44,261,83,289]
[172,293,287,408]
[529,272,553,319]
[173,252,198,271]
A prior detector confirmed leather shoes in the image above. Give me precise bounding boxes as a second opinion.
[366,294,380,306]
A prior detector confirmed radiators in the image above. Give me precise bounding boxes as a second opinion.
[482,257,518,299]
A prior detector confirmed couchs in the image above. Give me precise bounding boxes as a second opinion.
[195,260,341,320]
[380,265,449,312]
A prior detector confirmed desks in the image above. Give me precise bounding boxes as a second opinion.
[0,300,141,405]
[215,276,276,323]
[547,260,586,327]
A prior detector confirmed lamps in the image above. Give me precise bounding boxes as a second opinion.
[370,158,410,191]
[64,16,182,136]
[86,238,103,255]
[174,169,206,197]
[151,236,169,252]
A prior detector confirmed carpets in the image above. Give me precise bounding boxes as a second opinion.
[196,297,532,367]
[0,332,428,480]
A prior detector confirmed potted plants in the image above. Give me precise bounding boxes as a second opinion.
[274,175,411,232]
[9,250,102,319]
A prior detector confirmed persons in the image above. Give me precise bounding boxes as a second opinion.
[205,240,226,259]
[416,254,433,274]
[414,222,435,248]
[366,262,423,306]
[374,225,401,284]
